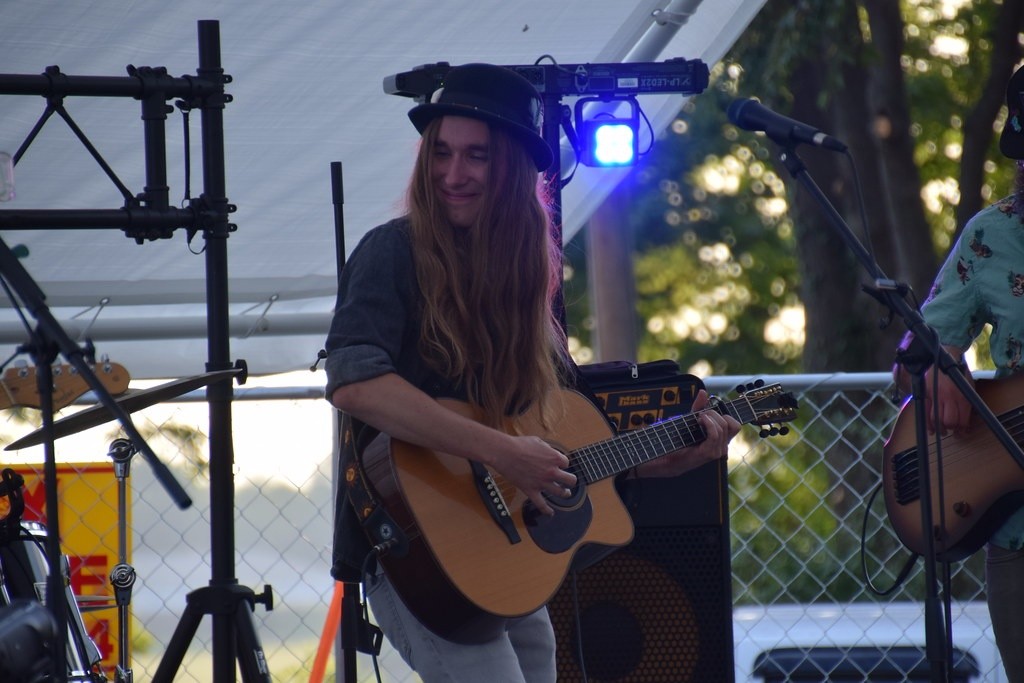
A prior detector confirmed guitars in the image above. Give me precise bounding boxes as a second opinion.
[356,381,799,652]
[3,342,131,409]
[879,357,1024,563]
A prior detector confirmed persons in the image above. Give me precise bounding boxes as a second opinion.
[324,64,742,683]
[892,67,1024,682]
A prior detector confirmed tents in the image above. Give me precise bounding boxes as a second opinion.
[0,0,770,380]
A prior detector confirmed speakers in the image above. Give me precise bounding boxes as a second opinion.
[546,359,733,683]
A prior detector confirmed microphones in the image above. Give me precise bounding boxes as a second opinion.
[726,98,847,154]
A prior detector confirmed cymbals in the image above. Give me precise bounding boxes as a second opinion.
[3,367,247,453]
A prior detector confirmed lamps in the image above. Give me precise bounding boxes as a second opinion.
[576,94,641,169]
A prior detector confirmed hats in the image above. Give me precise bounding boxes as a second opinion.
[999,66,1024,158]
[407,63,555,173]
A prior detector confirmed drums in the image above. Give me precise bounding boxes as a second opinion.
[0,519,103,674]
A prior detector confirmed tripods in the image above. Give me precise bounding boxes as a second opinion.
[156,18,282,682]
